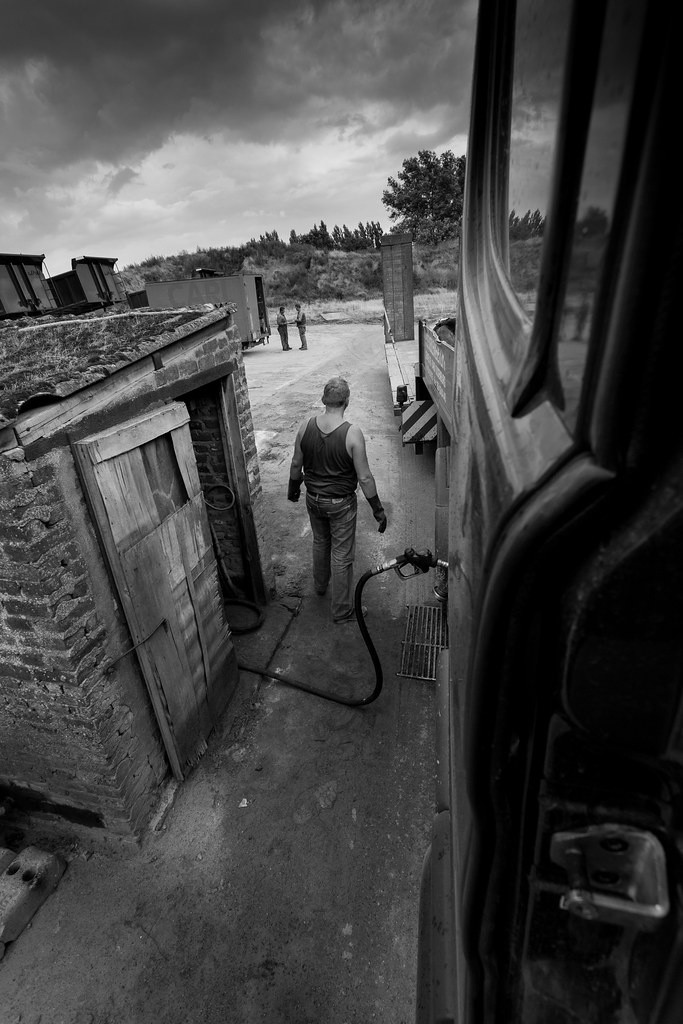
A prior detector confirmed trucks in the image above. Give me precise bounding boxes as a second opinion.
[143,273,273,350]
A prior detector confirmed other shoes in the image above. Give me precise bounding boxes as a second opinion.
[283,349,289,351]
[299,346,307,350]
[288,348,292,349]
[334,606,368,624]
[316,583,328,596]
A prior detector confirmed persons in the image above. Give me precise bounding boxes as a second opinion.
[277,304,308,351]
[288,378,387,624]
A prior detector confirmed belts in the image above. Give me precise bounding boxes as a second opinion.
[307,489,354,499]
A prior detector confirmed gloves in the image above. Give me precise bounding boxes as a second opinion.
[366,494,387,533]
[288,473,303,502]
